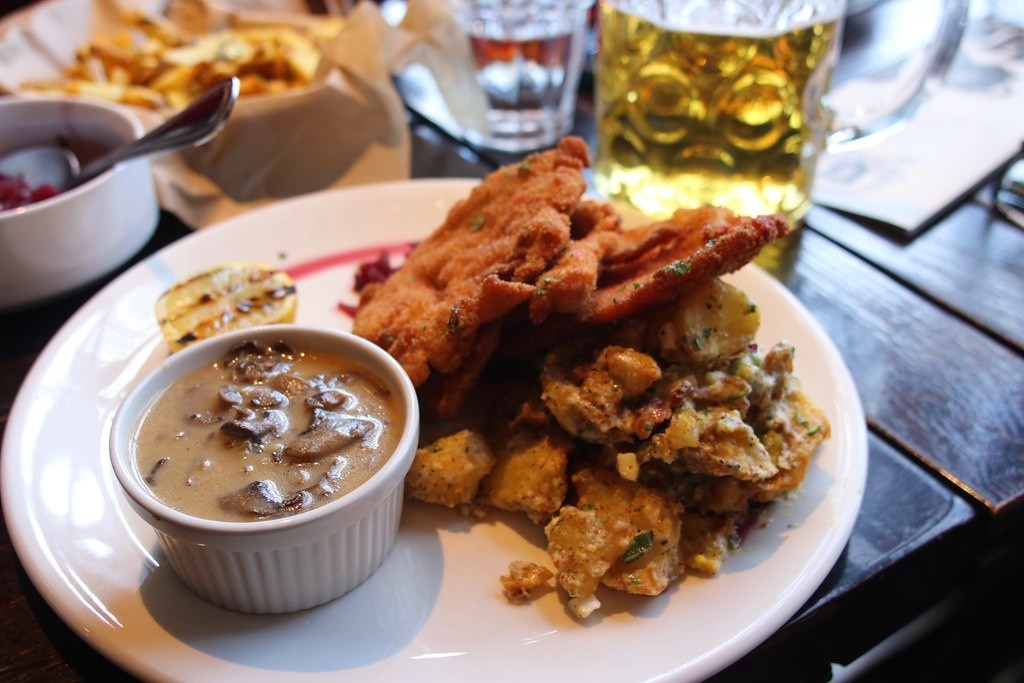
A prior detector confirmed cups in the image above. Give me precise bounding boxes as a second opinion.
[593,1,972,234]
[459,2,590,146]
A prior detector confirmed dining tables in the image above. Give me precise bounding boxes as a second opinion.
[0,0,1024,683]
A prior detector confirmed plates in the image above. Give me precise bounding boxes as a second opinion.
[3,177,868,680]
[1,1,381,114]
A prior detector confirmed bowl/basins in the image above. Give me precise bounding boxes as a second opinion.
[108,325,423,613]
[1,98,160,313]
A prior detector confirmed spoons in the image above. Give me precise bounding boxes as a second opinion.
[1,76,240,194]
